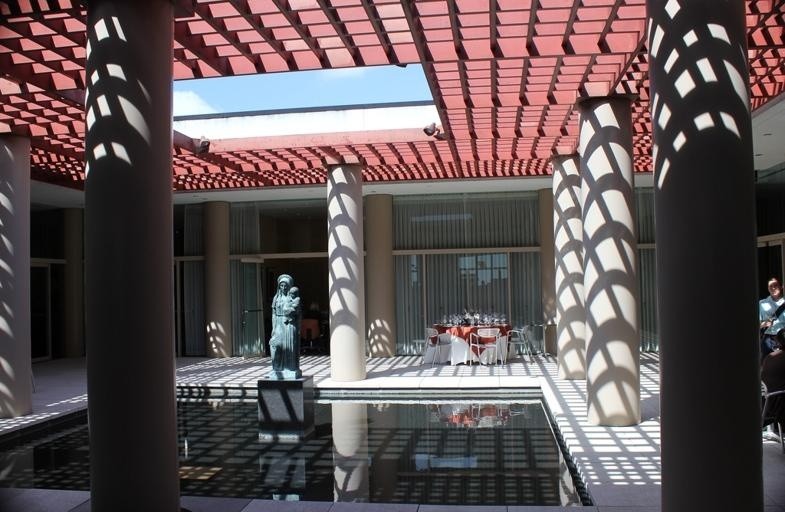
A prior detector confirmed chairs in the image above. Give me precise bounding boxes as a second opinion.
[422,326,534,369]
[301,319,321,355]
[429,404,510,428]
[761,381,785,454]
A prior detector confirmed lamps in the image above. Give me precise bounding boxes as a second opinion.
[195,135,210,157]
[424,122,445,142]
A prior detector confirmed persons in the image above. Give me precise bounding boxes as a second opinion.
[310,301,323,336]
[759,278,785,434]
[269,274,301,371]
